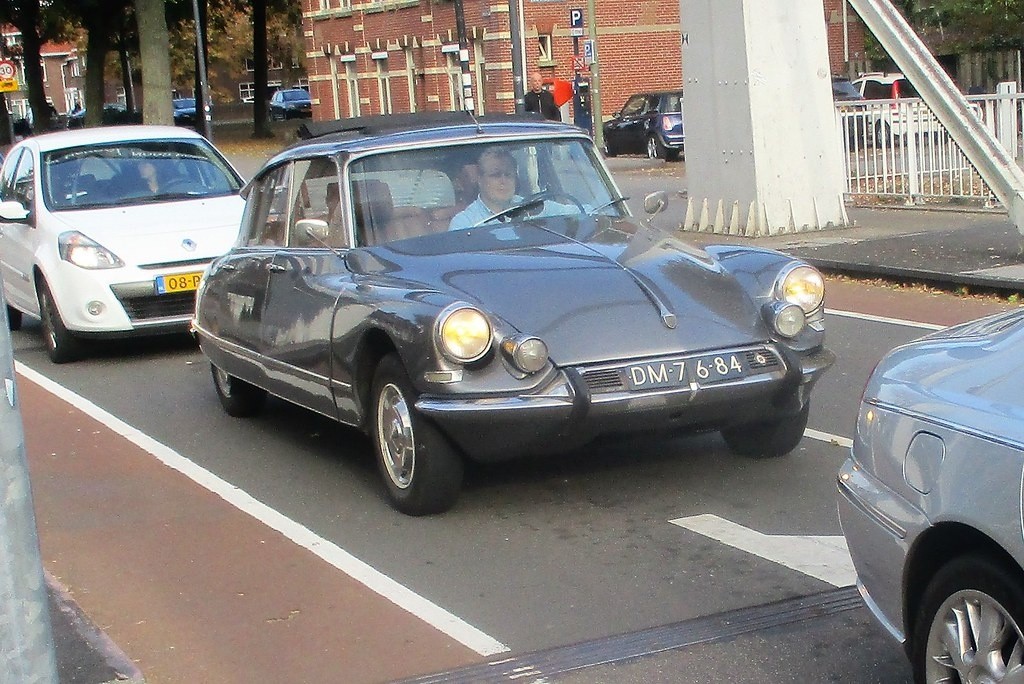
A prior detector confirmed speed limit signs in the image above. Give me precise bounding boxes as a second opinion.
[0,60,17,82]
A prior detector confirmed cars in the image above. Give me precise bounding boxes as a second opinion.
[188,122,834,519]
[836,304,1024,683]
[71,104,127,121]
[602,89,685,161]
[269,88,313,123]
[173,97,198,126]
[0,125,277,366]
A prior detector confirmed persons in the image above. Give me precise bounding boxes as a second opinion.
[138,155,164,194]
[448,146,598,231]
[524,73,561,122]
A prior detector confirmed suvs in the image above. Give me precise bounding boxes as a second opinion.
[851,72,947,149]
[828,72,869,153]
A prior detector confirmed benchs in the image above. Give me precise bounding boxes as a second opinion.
[263,206,468,248]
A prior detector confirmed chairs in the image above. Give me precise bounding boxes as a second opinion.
[326,178,400,249]
[450,164,521,221]
